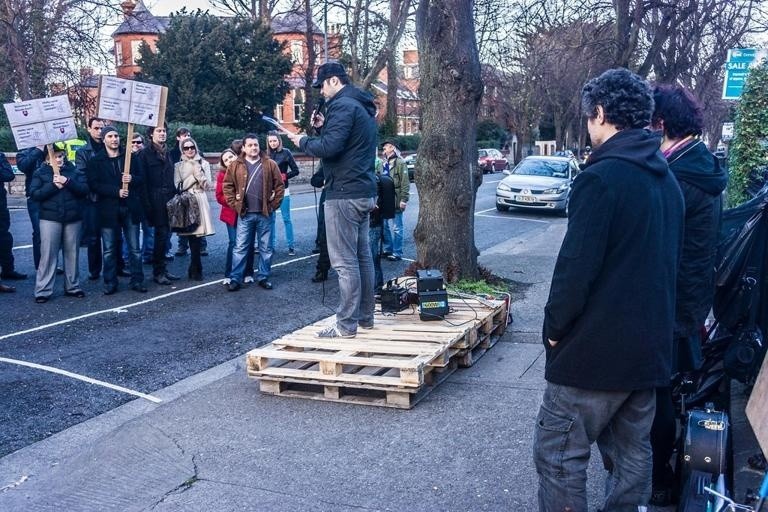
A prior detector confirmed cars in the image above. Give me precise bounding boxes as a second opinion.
[494,157,581,218]
[552,150,574,159]
[406,153,418,183]
[477,148,510,175]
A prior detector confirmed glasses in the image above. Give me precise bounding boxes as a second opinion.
[132,141,143,144]
[267,130,278,135]
[183,145,196,150]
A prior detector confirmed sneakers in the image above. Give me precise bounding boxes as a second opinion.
[254,247,275,254]
[312,247,321,254]
[289,249,297,256]
[222,275,273,292]
[373,294,383,313]
[311,319,374,339]
[381,250,402,261]
[312,270,328,282]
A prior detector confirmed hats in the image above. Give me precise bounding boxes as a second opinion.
[381,136,399,149]
[99,125,119,141]
[40,143,66,162]
[311,61,349,88]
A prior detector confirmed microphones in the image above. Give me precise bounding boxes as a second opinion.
[313,96,324,121]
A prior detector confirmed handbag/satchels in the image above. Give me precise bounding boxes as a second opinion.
[165,190,202,234]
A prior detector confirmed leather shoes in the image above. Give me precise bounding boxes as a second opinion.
[36,295,51,303]
[0,270,28,281]
[64,290,85,298]
[0,284,16,293]
[132,283,148,293]
[104,285,117,295]
[56,246,212,281]
[153,275,173,285]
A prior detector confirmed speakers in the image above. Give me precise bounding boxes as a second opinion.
[416,269,443,304]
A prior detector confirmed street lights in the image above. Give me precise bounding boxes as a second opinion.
[528,122,535,156]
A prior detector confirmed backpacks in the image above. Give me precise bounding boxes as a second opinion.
[379,175,395,219]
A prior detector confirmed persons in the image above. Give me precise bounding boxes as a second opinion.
[216,134,284,292]
[531,68,687,512]
[15,118,218,303]
[592,382,679,511]
[309,157,331,282]
[288,61,377,337]
[266,129,298,256]
[647,80,731,376]
[583,146,592,160]
[0,152,27,292]
[378,138,410,260]
[369,158,384,299]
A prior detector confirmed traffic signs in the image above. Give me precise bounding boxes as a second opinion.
[725,49,755,99]
